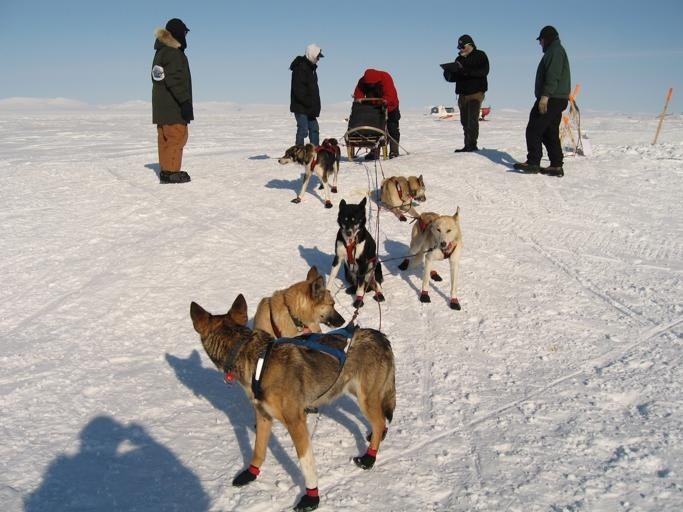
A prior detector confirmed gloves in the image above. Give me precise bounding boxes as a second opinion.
[539,96,549,114]
[181,102,194,124]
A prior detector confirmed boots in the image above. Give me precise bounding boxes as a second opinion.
[540,165,564,177]
[159,171,191,184]
[365,148,379,161]
[389,135,400,159]
[513,160,540,174]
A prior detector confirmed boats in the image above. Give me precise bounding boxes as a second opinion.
[423,104,493,124]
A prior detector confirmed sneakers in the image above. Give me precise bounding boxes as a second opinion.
[455,145,478,152]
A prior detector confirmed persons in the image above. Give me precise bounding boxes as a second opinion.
[354,68,400,160]
[443,34,490,153]
[289,43,325,147]
[512,26,572,177]
[150,18,194,183]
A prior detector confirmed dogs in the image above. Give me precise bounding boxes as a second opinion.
[254,266,345,414]
[325,197,385,308]
[398,207,462,310]
[191,294,396,512]
[278,138,340,208]
[381,175,426,221]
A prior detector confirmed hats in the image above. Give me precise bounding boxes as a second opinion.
[457,35,474,49]
[536,26,559,52]
[165,18,190,35]
[317,49,325,57]
